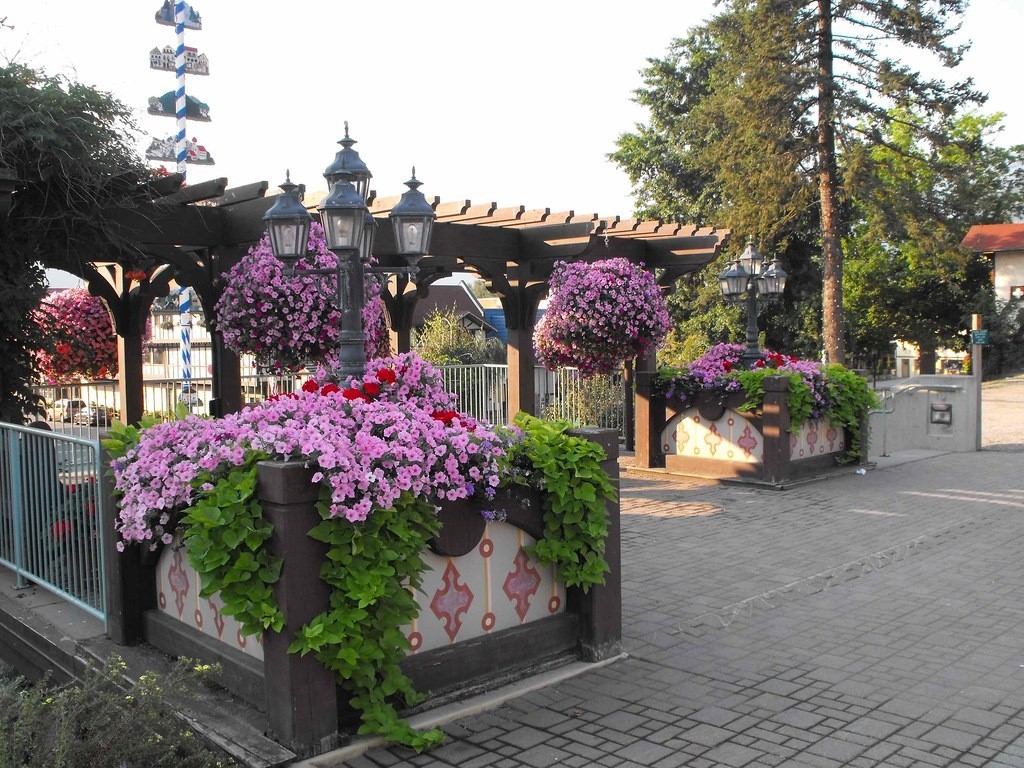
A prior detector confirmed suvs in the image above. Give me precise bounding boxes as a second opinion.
[46,398,86,423]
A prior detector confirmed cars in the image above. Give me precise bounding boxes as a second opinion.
[74,405,116,426]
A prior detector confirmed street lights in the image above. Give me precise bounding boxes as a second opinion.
[260,120,442,396]
[715,232,789,367]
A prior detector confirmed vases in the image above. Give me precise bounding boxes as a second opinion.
[72,541,94,572]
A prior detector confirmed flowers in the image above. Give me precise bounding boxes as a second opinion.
[42,477,97,546]
[29,288,154,386]
[532,259,674,377]
[210,220,391,375]
